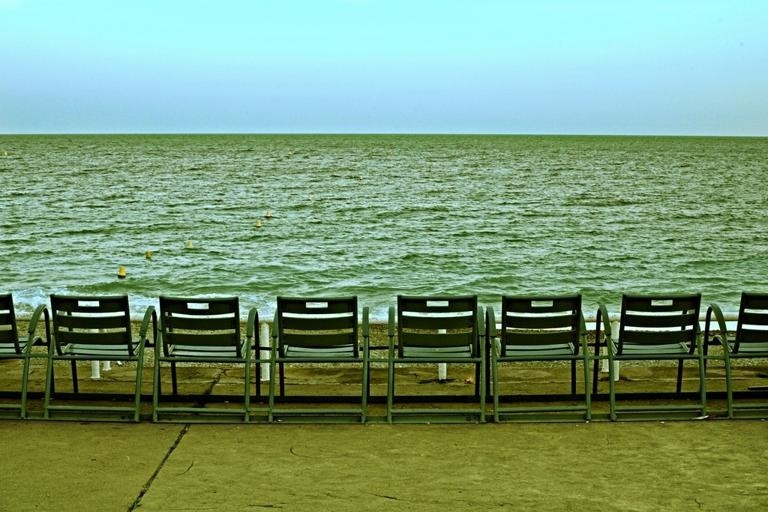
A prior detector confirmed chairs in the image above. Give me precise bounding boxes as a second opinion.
[702,292,768,421]
[0,293,50,422]
[486,294,592,425]
[387,294,487,425]
[268,295,371,425]
[152,293,260,427]
[45,294,158,425]
[593,294,707,418]
